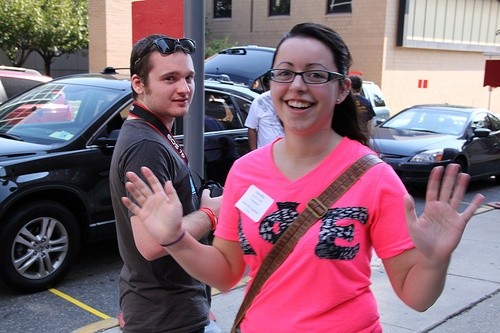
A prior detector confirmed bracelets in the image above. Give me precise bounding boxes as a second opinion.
[160,229,186,247]
[198,207,217,230]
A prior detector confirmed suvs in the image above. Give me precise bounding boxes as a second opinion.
[202,43,277,95]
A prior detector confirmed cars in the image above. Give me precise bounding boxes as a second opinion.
[359,80,391,128]
[0,67,263,296]
[0,64,72,127]
[367,102,500,196]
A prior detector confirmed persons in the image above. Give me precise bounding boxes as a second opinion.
[245,89,285,151]
[110,33,225,333]
[348,76,376,145]
[122,22,485,333]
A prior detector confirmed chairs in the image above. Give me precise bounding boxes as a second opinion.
[417,114,439,128]
[440,118,454,130]
[90,101,123,138]
[205,101,231,130]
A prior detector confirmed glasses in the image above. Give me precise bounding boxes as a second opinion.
[134,38,196,65]
[267,66,344,85]
